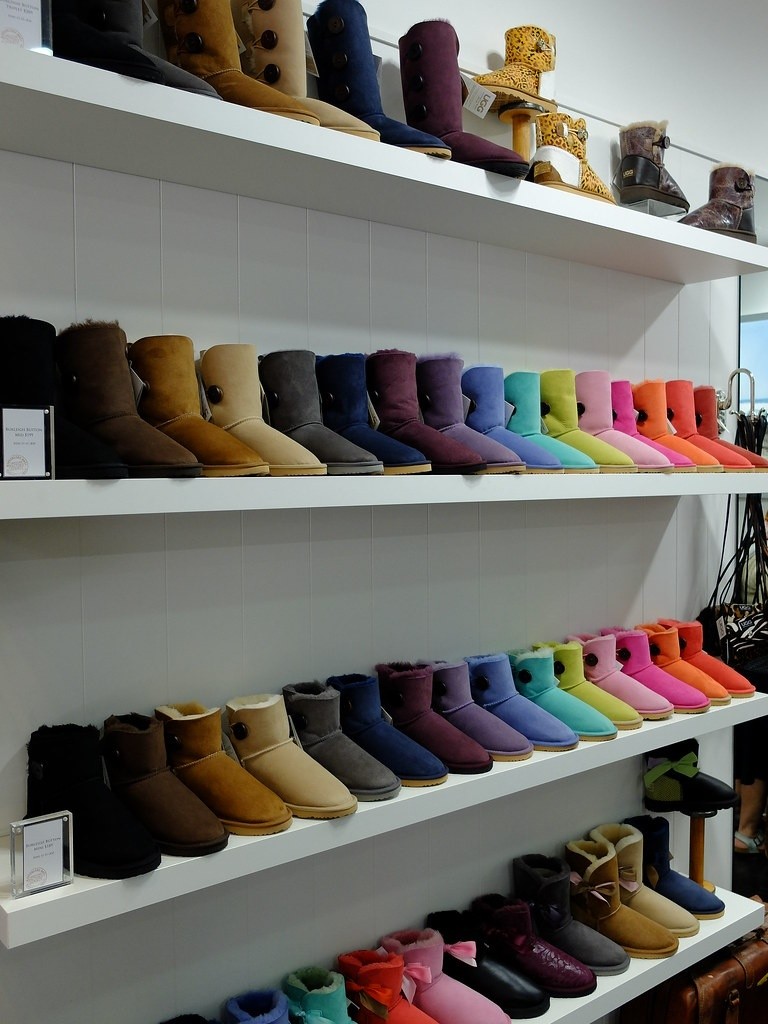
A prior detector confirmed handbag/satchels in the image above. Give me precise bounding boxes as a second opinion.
[695,603,768,659]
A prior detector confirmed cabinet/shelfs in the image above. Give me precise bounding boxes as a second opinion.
[0,0,767,1024]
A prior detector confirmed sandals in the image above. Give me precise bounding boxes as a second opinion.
[735,831,768,856]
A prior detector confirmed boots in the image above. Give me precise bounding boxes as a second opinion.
[676,161,757,244]
[469,26,558,124]
[158,814,726,1024]
[0,315,768,478]
[24,618,756,879]
[619,120,690,213]
[534,113,618,205]
[52,0,530,180]
[642,738,740,814]
